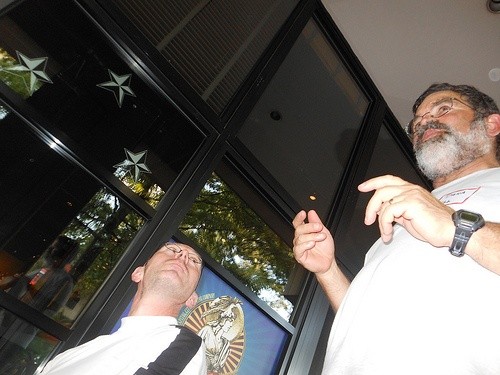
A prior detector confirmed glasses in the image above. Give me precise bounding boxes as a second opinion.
[408,97,488,134]
[165,239,203,265]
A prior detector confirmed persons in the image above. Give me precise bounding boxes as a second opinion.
[38,242,208,375]
[292,83,500,375]
[0,234,80,375]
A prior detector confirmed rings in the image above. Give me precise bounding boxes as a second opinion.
[389,199,394,204]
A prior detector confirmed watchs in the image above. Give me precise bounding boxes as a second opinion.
[449,209,486,257]
